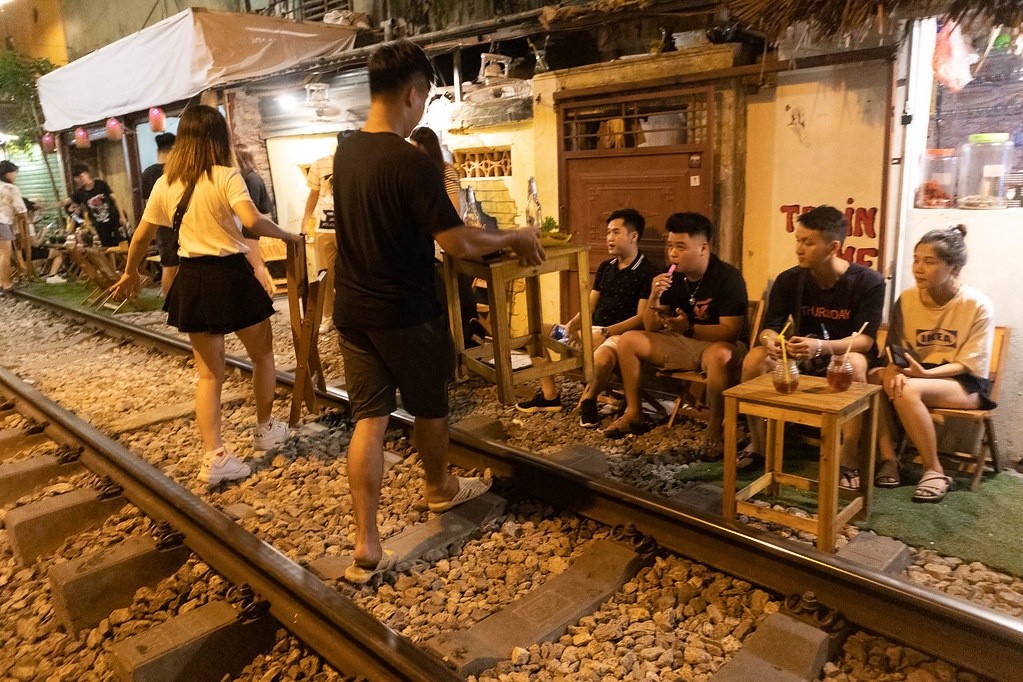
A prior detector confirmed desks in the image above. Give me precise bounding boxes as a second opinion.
[440,243,592,406]
[723,372,882,554]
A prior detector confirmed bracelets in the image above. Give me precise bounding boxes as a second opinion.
[812,339,821,359]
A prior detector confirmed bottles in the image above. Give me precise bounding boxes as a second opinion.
[826,354,853,392]
[914,132,1015,209]
[462,185,485,231]
[524,175,542,230]
[772,358,800,394]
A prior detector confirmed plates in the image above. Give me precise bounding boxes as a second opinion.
[540,231,572,246]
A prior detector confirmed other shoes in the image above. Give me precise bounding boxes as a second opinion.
[46,275,67,284]
[698,430,724,461]
[603,419,645,437]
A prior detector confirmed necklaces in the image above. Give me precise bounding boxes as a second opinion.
[681,272,704,305]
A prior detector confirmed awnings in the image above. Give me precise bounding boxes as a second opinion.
[36,8,376,132]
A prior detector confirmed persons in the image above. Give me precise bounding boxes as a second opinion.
[596,210,749,462]
[110,104,302,483]
[868,224,997,501]
[302,130,357,333]
[0,160,131,292]
[332,40,547,583]
[735,206,886,490]
[143,134,178,298]
[410,126,485,359]
[234,151,272,299]
[515,209,660,427]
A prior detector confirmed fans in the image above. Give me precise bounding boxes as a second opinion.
[306,83,342,119]
[463,53,531,107]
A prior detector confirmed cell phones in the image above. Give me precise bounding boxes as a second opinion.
[649,305,675,317]
[547,325,582,349]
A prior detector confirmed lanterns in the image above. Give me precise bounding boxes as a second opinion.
[149,106,165,134]
[76,127,90,150]
[106,117,124,142]
[42,132,57,155]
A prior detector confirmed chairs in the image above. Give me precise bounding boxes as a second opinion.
[898,327,1011,493]
[656,300,765,427]
[286,234,327,428]
[69,249,150,314]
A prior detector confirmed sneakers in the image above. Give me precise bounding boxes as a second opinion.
[579,399,601,427]
[515,388,562,414]
[197,445,251,484]
[253,415,289,450]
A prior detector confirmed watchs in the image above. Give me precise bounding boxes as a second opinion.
[683,321,695,338]
[600,327,611,339]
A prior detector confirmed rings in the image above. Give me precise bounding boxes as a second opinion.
[796,353,802,360]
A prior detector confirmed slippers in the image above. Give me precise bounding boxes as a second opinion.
[876,460,906,487]
[838,464,860,490]
[912,472,954,502]
[736,450,766,473]
[428,475,492,512]
[344,548,398,583]
